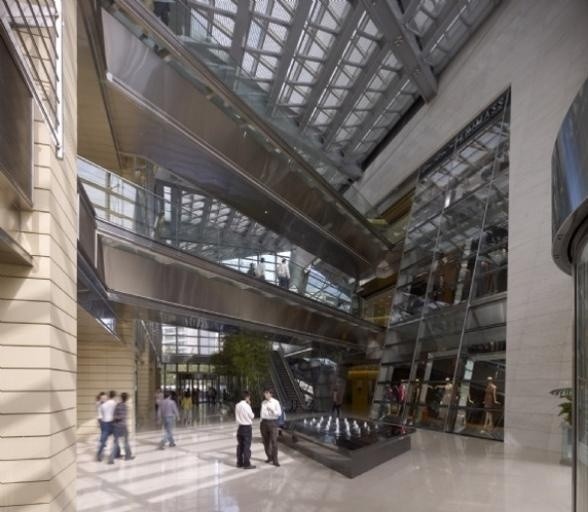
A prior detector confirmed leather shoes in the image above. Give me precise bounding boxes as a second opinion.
[238,458,280,469]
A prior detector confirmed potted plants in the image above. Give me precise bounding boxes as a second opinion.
[548,386,572,466]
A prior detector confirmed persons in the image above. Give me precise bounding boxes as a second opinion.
[259,388,282,466]
[95,386,228,464]
[383,377,499,432]
[255,258,265,281]
[331,388,341,417]
[247,263,255,275]
[235,391,256,469]
[277,259,290,289]
